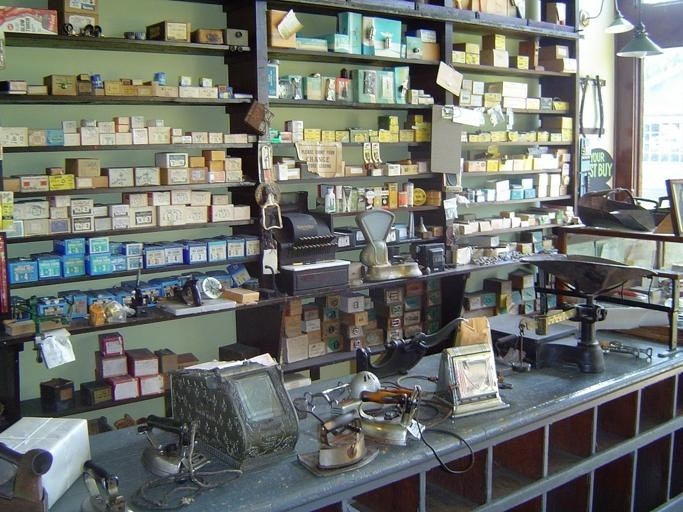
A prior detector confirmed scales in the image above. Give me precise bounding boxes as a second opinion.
[511,255,657,376]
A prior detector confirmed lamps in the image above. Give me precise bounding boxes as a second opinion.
[617,1,664,59]
[578,0,636,34]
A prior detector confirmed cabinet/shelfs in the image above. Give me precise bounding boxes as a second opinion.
[0,0,581,433]
[0,304,683,512]
